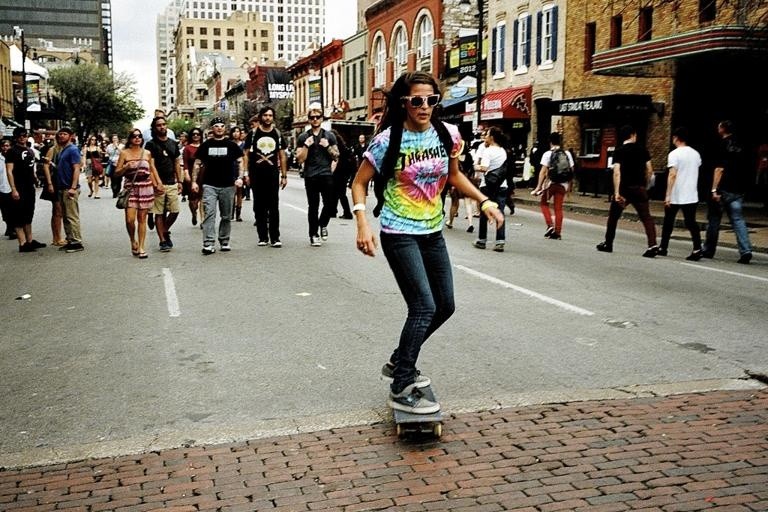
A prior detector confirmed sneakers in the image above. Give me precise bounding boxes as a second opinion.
[310,235,321,245]
[493,244,505,251]
[596,242,613,252]
[159,230,173,252]
[387,384,440,414]
[257,240,268,246]
[221,243,231,251]
[737,253,752,264]
[379,363,431,388]
[321,226,328,241]
[544,224,554,237]
[19,242,37,252]
[202,245,216,252]
[270,240,282,246]
[31,240,47,248]
[642,247,667,257]
[471,240,486,249]
[51,239,84,253]
[686,249,714,261]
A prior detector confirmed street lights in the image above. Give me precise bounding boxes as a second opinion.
[18,29,40,124]
[459,0,485,128]
[308,42,325,116]
[229,94,239,125]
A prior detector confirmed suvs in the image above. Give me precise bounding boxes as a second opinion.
[280,125,305,176]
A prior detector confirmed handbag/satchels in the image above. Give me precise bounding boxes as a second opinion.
[105,160,112,177]
[116,188,130,208]
[92,160,103,177]
[485,159,507,188]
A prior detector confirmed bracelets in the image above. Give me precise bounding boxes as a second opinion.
[711,189,717,193]
[614,193,619,196]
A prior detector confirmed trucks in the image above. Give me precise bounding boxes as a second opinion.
[301,120,376,148]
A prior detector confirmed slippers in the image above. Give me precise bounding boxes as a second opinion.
[139,253,148,259]
[132,242,139,255]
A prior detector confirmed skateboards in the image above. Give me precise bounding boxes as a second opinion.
[393,377,444,438]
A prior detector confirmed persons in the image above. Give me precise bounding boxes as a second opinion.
[657,127,701,260]
[701,119,754,266]
[82,110,288,261]
[285,102,369,247]
[595,124,661,257]
[529,132,575,241]
[0,128,85,253]
[350,70,506,416]
[446,127,515,252]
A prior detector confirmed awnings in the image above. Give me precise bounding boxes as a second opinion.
[478,85,532,120]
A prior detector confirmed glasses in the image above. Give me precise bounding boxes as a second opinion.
[400,94,440,109]
[16,134,27,138]
[192,133,200,136]
[131,134,143,138]
[213,124,225,128]
[310,115,321,120]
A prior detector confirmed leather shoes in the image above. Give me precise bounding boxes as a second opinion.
[445,220,453,228]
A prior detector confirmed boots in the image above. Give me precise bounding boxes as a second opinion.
[236,206,242,221]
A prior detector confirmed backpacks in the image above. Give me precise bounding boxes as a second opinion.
[547,148,573,183]
